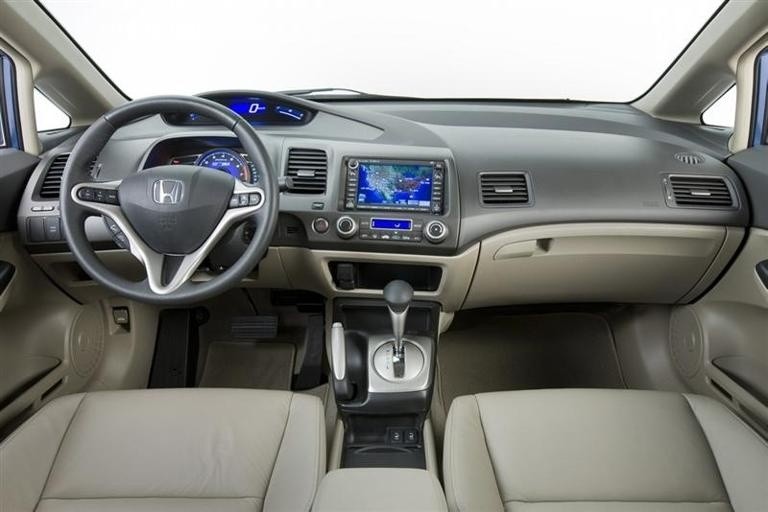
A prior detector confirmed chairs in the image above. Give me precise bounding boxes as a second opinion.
[441,387,768,512]
[0,387,324,512]
